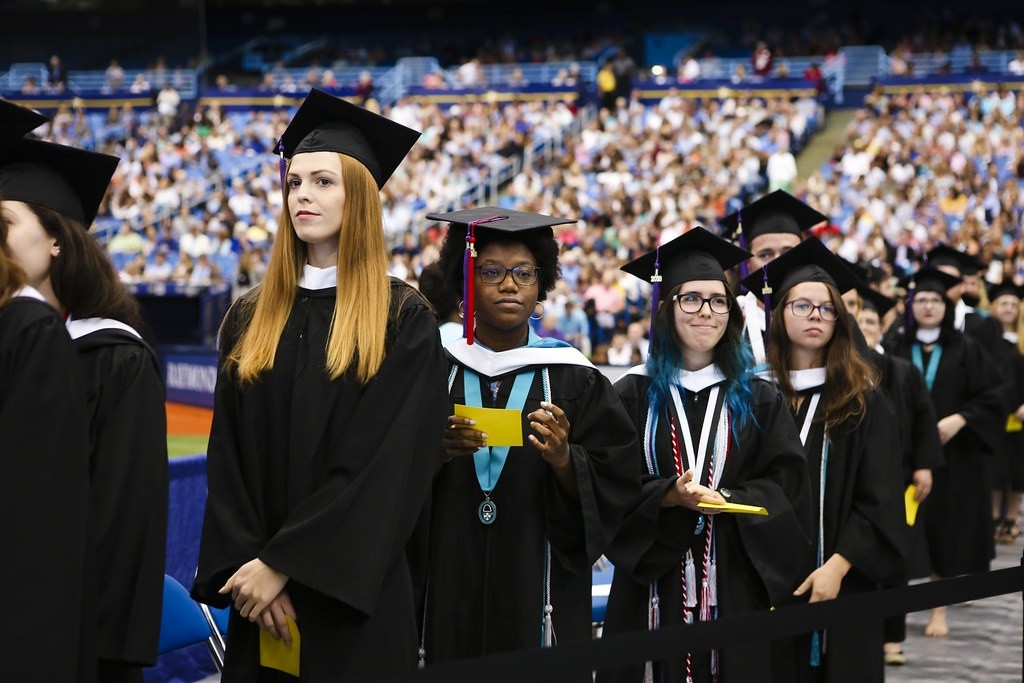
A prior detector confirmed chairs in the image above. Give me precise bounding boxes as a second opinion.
[194,565,231,654]
[590,557,614,628]
[156,573,224,675]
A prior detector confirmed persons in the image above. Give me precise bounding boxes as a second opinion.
[0,40,1024,368]
[604,226,815,683]
[418,208,642,683]
[190,88,448,683]
[732,189,1024,683]
[0,99,170,683]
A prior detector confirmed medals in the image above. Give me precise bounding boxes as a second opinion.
[478,498,496,526]
[695,516,703,534]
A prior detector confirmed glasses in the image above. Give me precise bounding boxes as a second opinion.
[783,298,841,322]
[667,293,734,314]
[473,265,541,286]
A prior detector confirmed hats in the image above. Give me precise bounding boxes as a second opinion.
[926,242,989,275]
[619,226,754,354]
[894,264,964,327]
[425,204,578,345]
[985,275,1024,303]
[0,97,121,232]
[271,87,422,192]
[739,235,866,340]
[866,284,899,322]
[717,188,829,241]
